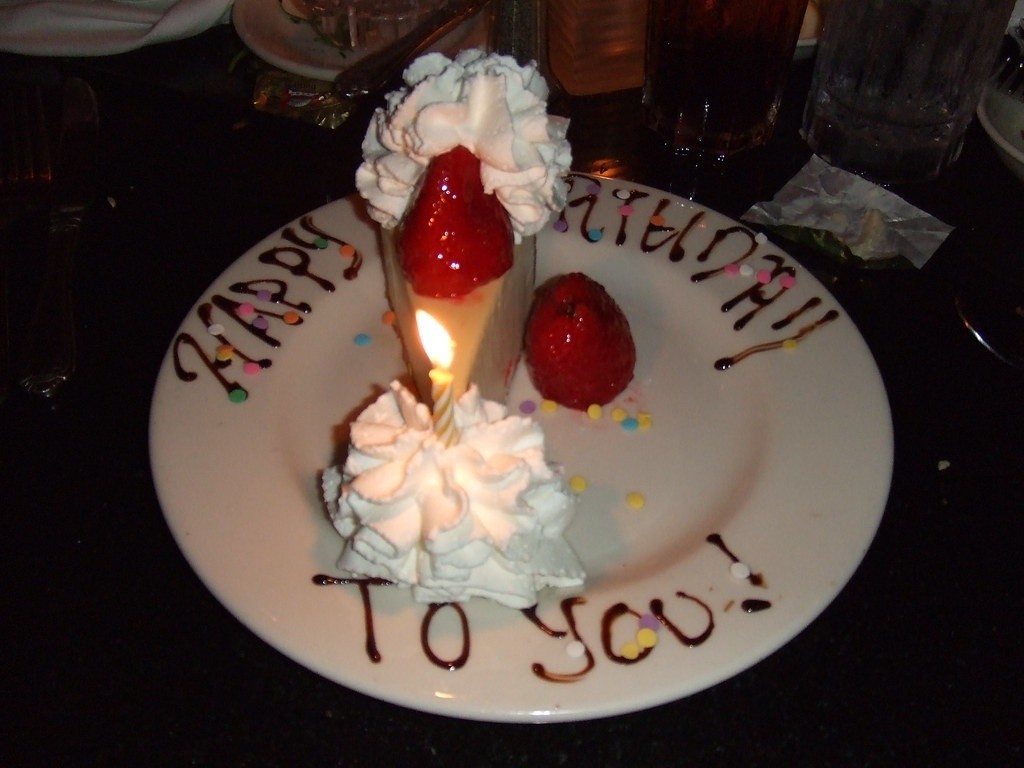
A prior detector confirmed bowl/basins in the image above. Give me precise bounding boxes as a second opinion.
[976,83,1024,184]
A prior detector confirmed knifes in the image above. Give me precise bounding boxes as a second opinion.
[25,77,100,392]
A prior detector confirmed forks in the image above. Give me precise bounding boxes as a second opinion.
[1,80,65,394]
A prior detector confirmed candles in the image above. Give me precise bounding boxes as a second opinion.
[413,309,460,447]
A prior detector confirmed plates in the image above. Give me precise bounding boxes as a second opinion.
[232,0,496,85]
[150,171,897,727]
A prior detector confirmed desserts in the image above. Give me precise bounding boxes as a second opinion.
[320,49,637,609]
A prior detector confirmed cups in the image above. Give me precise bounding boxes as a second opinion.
[798,0,1018,175]
[641,0,810,166]
